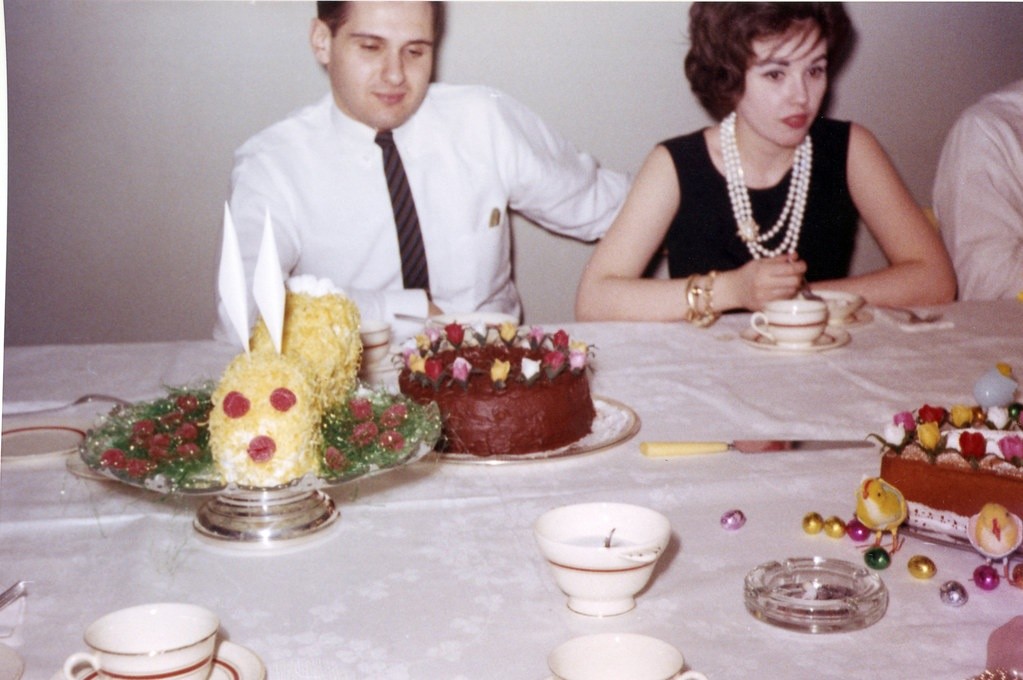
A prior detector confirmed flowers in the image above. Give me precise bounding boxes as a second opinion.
[861,403,1023,469]
[388,318,599,393]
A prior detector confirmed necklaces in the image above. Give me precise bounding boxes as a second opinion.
[719,106,813,263]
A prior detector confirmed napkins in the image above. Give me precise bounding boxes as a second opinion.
[870,305,954,333]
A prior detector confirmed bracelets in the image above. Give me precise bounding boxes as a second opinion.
[681,272,720,328]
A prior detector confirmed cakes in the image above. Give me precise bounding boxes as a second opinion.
[864,400,1023,559]
[397,319,598,455]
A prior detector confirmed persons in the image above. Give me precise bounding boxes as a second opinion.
[932,79,1023,298]
[573,2,956,323]
[212,0,668,352]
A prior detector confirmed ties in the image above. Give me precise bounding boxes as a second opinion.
[373,130,432,303]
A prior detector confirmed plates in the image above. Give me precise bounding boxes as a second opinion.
[358,348,405,376]
[1,421,93,462]
[826,312,872,328]
[737,323,854,351]
[0,639,25,680]
[49,638,264,680]
[422,396,640,467]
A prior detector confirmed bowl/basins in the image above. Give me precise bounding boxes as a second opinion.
[797,290,866,321]
[425,313,520,335]
[533,501,671,620]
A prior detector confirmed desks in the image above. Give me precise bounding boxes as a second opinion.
[0,300,1023,680]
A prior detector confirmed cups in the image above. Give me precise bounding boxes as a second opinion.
[358,320,390,369]
[751,299,829,345]
[550,631,705,680]
[63,601,223,680]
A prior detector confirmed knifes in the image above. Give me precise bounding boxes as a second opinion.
[641,440,875,453]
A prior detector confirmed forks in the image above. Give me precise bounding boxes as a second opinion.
[0,581,28,610]
[881,303,946,324]
[783,254,826,300]
[3,392,132,419]
